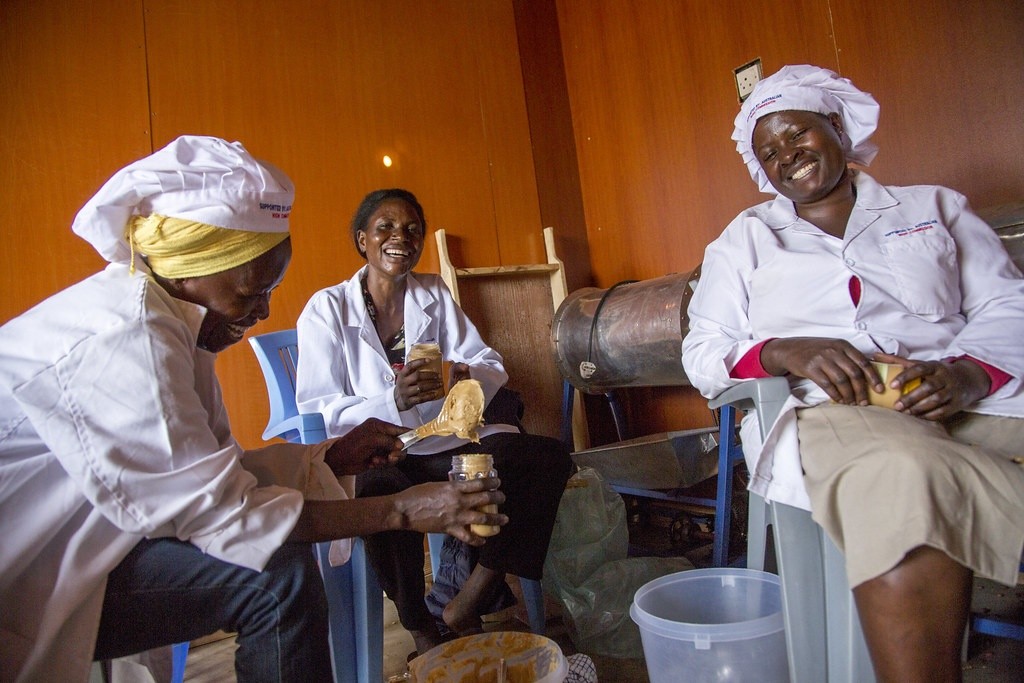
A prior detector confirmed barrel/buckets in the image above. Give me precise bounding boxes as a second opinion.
[629,568,791,683]
[410,631,570,683]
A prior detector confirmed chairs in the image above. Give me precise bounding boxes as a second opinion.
[247,329,546,683]
[711,374,1024,683]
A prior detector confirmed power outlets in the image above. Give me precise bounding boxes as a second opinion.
[736,65,761,101]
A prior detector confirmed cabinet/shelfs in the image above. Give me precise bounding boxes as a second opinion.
[561,378,747,568]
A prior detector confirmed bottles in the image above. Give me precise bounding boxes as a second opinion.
[448,454,500,537]
[407,342,445,401]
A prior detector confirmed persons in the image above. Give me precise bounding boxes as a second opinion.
[681,65,1024,683]
[0,134,509,683]
[294,188,573,661]
